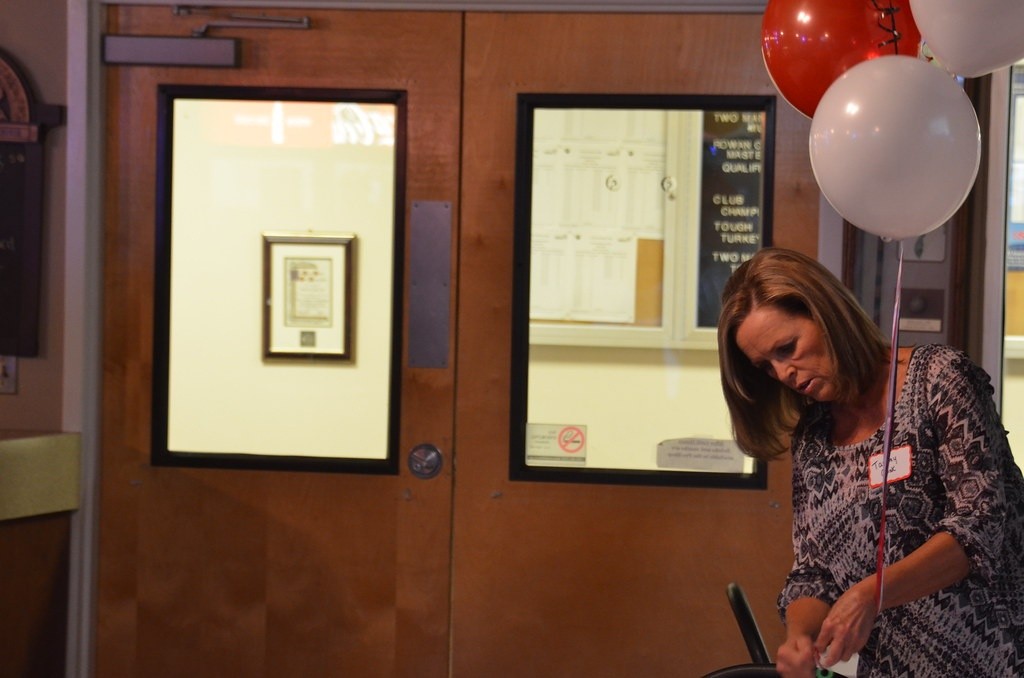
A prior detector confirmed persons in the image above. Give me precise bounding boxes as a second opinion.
[717,246,1024,678]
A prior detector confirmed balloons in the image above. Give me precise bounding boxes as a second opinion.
[760,0,1024,239]
